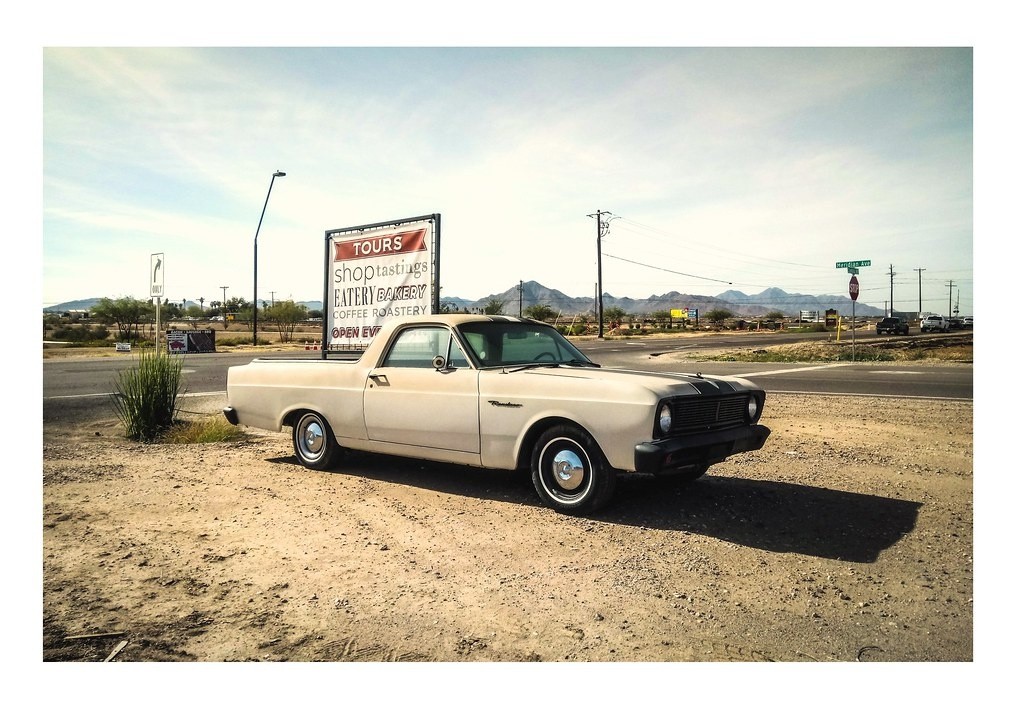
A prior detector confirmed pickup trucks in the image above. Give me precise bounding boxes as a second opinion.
[223,314,771,515]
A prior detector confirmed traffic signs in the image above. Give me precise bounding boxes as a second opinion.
[836,259,871,275]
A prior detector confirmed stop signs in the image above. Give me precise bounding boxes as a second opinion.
[849,276,859,301]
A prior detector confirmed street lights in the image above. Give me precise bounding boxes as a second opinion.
[253,170,287,347]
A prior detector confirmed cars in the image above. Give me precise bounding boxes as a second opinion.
[877,317,909,335]
[920,315,949,332]
[946,317,973,327]
